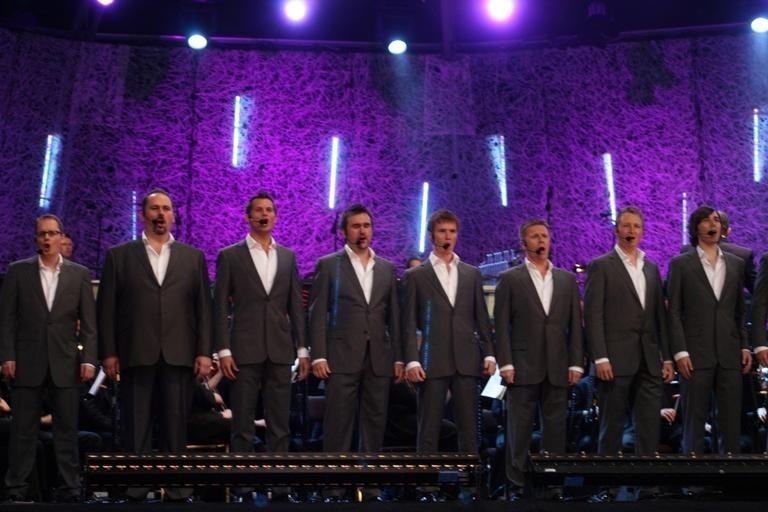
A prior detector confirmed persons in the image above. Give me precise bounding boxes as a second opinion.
[310,202,408,503]
[52,233,79,262]
[574,204,678,503]
[0,251,768,462]
[212,189,310,503]
[491,215,585,502]
[396,206,495,504]
[666,204,754,498]
[98,186,214,503]
[679,208,755,298]
[0,211,104,503]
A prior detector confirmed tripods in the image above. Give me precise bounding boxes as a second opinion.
[491,398,512,501]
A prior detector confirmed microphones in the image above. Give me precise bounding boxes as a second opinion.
[525,246,540,254]
[32,244,44,255]
[145,216,158,224]
[251,219,265,224]
[697,231,714,235]
[348,239,361,245]
[433,242,448,249]
[619,236,630,241]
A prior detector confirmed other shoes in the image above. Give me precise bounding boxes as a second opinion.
[4,486,767,502]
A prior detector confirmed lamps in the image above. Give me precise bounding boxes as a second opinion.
[186,4,212,51]
[384,10,408,54]
